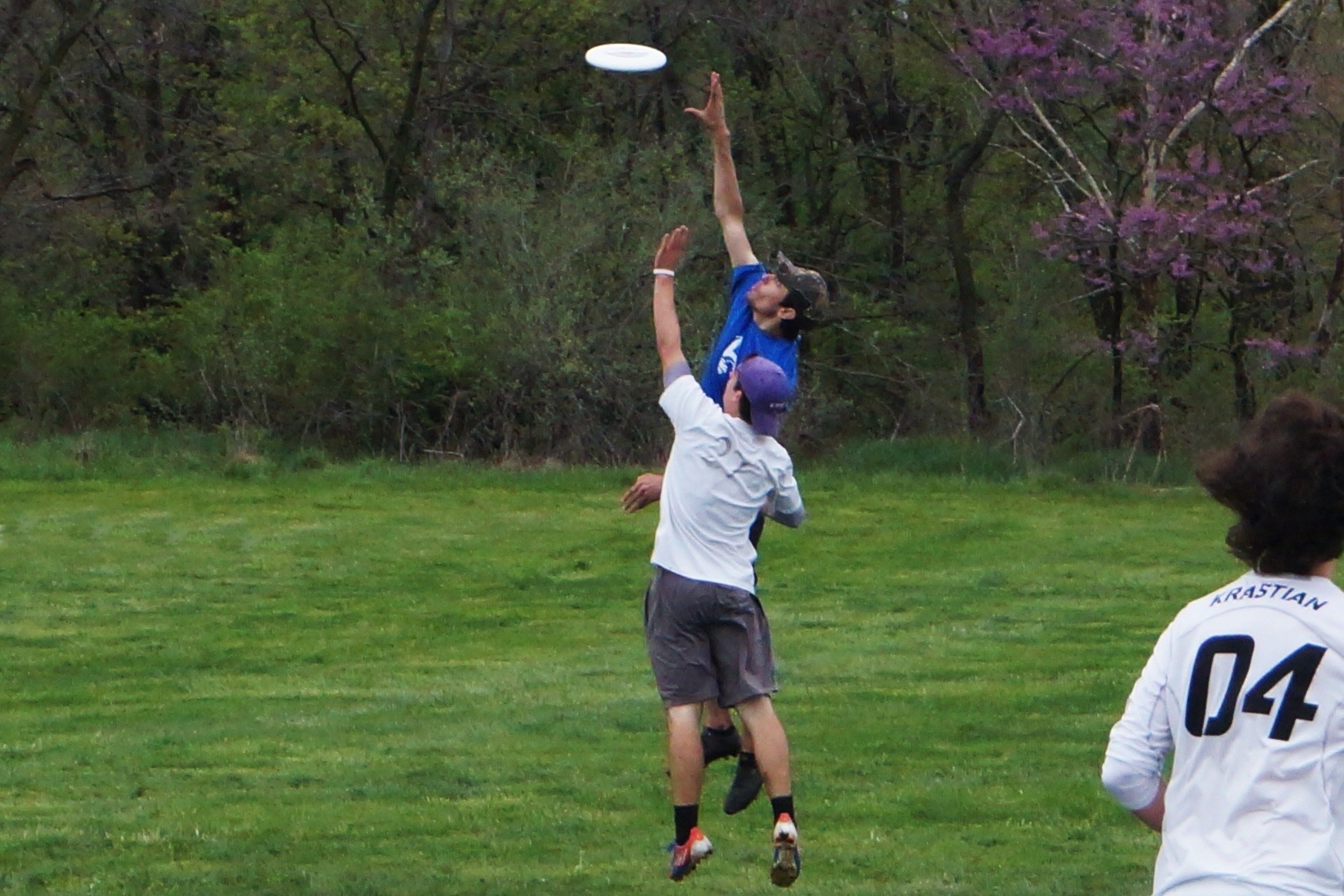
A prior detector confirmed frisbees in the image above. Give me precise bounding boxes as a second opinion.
[584,42,669,74]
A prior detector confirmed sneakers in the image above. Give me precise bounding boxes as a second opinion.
[724,753,763,815]
[770,813,799,887]
[670,827,713,879]
[665,726,743,778]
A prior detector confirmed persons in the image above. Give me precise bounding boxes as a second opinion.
[620,70,828,816]
[643,225,806,888]
[1100,394,1344,896]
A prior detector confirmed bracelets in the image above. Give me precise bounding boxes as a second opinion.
[652,268,674,276]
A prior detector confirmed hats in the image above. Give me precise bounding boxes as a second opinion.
[775,250,829,323]
[739,356,790,438]
